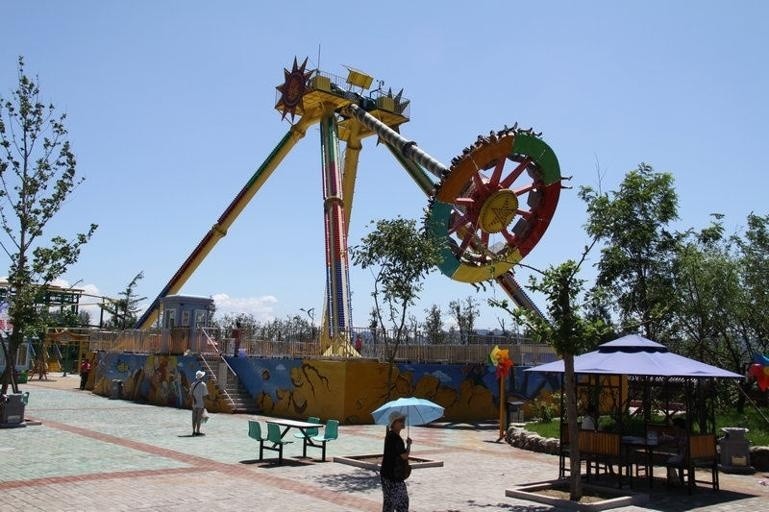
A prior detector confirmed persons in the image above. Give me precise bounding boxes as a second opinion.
[355,335,363,354]
[189,370,208,437]
[79,358,92,390]
[379,411,413,512]
[665,417,690,487]
[232,320,242,358]
[417,118,575,295]
[580,404,600,431]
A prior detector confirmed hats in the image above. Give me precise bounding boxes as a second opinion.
[195,371,205,380]
[389,411,408,426]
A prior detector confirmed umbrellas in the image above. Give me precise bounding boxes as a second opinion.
[521,332,747,474]
[370,396,446,439]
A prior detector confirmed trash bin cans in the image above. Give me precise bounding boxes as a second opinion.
[719,427,756,473]
[2,393,25,425]
[508,401,524,422]
[110,380,123,398]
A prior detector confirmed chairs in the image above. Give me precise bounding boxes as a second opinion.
[557,420,721,496]
[0,390,30,428]
[248,416,339,464]
